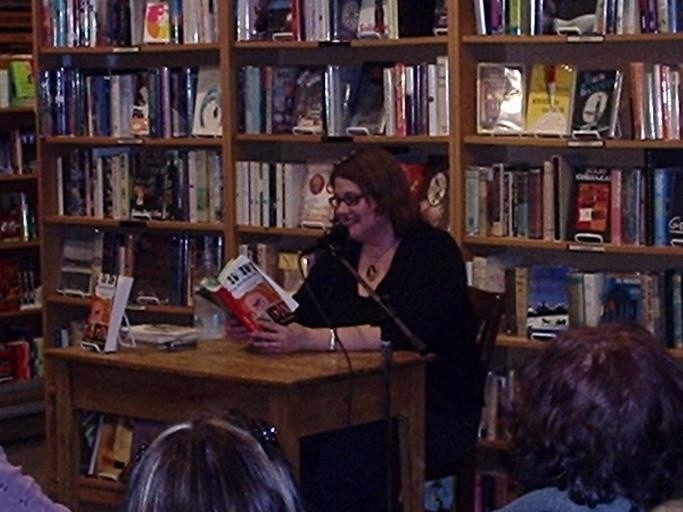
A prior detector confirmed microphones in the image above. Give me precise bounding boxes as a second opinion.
[301,225,351,255]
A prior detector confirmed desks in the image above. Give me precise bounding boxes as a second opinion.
[41,332,440,512]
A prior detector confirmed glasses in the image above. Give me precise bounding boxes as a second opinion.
[327,190,373,211]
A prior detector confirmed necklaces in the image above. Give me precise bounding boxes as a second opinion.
[360,237,399,282]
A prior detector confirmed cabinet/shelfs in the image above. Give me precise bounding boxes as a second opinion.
[32,1,681,512]
[1,0,48,435]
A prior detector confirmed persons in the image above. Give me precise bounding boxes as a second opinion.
[223,148,484,511]
[118,403,304,510]
[0,438,75,510]
[486,321,682,510]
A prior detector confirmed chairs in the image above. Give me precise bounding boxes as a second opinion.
[377,288,505,512]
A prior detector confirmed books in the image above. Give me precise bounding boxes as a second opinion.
[411,457,523,510]
[76,458,168,483]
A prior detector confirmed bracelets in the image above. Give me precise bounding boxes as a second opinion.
[327,328,337,351]
